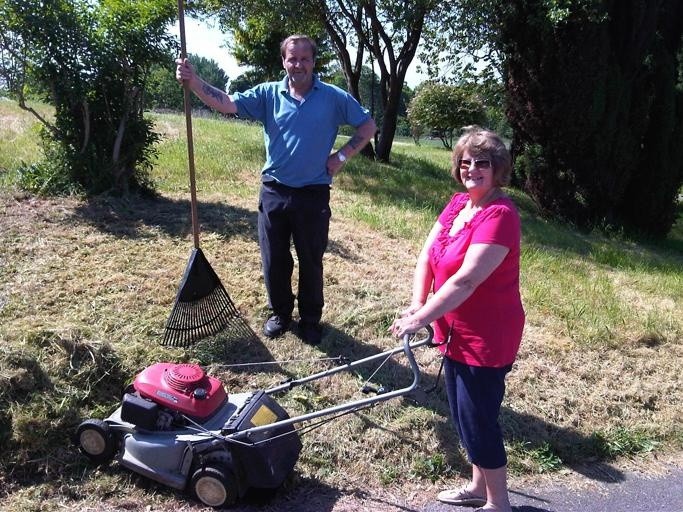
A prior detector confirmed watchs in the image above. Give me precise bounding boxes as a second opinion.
[336,149,350,163]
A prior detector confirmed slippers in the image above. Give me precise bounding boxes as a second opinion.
[438,488,487,507]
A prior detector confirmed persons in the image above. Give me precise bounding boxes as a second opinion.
[387,123,526,512]
[175,34,379,336]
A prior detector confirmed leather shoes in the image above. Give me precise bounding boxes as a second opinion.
[263,314,292,337]
[298,319,321,344]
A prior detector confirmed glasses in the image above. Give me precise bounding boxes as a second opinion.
[457,159,491,169]
